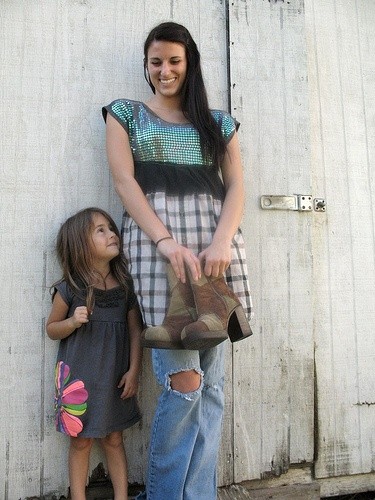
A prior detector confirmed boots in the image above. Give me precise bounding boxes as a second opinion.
[141,263,199,349]
[179,259,254,349]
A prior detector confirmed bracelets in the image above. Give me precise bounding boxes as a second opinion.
[155,236,174,249]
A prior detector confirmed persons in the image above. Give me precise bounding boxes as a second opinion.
[99,23,251,500]
[44,206,144,499]
